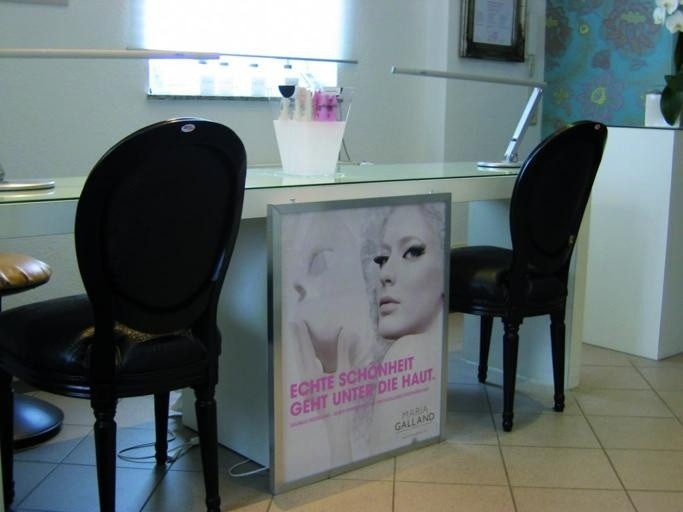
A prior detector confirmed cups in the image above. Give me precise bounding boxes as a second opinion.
[265,86,357,178]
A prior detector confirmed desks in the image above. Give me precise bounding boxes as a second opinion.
[0,160,592,498]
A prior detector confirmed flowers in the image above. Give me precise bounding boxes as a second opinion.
[651,0,683,37]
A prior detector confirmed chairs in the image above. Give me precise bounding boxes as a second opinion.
[0,116,250,510]
[445,118,607,435]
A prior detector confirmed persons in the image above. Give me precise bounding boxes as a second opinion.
[290,204,447,468]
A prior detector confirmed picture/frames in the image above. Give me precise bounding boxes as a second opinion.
[458,0,528,64]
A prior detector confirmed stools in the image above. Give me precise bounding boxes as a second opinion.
[1,252,65,448]
